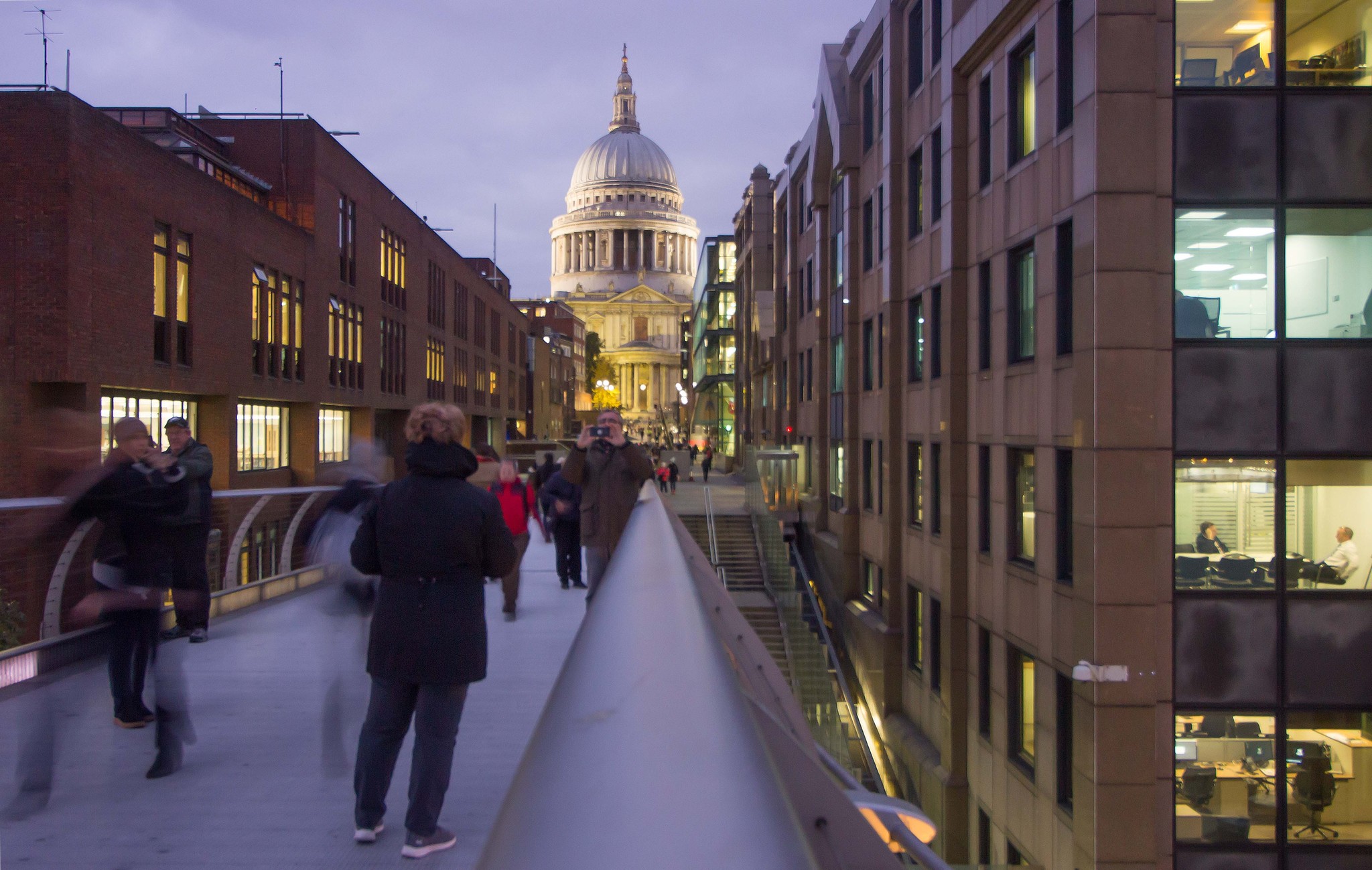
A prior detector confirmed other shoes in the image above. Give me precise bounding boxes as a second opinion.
[503,600,517,620]
[190,628,208,644]
[562,583,570,589]
[574,580,589,589]
[164,624,187,639]
[146,745,181,775]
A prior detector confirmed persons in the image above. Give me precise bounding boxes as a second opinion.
[489,459,536,622]
[562,409,653,614]
[1297,526,1359,582]
[465,443,502,492]
[705,447,712,470]
[700,455,711,483]
[688,445,699,466]
[657,462,670,493]
[538,468,588,590]
[527,451,567,542]
[40,416,212,779]
[316,437,396,619]
[623,415,687,480]
[1196,520,1229,553]
[349,401,517,861]
[667,457,678,495]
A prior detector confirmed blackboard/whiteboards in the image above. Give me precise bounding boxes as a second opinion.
[1286,258,1328,319]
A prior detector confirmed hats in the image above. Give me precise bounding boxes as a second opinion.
[661,462,666,467]
[671,457,675,463]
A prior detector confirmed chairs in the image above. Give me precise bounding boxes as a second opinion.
[1193,297,1231,338]
[1286,755,1339,839]
[1236,723,1262,738]
[1174,544,1346,590]
[1175,59,1217,86]
[1175,766,1218,814]
[1201,815,1250,844]
[1199,715,1236,738]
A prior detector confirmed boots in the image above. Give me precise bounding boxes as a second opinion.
[672,489,675,494]
[131,605,161,721]
[103,605,145,728]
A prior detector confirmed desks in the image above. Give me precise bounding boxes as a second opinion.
[1176,552,1314,588]
[1236,65,1366,86]
[1175,761,1355,838]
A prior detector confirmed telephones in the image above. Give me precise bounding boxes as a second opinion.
[1240,756,1258,771]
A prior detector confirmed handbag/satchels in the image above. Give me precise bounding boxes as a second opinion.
[308,499,382,588]
[660,472,664,482]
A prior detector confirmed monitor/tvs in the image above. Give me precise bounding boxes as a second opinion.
[1175,741,1197,763]
[1244,741,1274,766]
[1286,741,1306,764]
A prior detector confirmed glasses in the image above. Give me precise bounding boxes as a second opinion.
[597,418,623,426]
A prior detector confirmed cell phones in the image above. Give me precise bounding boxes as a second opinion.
[589,426,610,437]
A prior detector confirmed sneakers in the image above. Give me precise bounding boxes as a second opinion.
[401,825,457,858]
[354,817,384,838]
